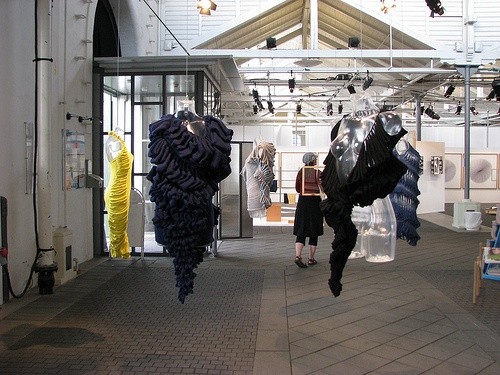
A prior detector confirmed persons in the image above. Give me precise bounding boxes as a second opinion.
[291,151,328,269]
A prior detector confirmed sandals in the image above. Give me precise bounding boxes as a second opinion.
[295,257,318,268]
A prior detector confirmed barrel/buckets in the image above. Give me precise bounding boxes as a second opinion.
[464,212,482,230]
[453,200,480,228]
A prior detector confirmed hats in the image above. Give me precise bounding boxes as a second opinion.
[302,152,319,164]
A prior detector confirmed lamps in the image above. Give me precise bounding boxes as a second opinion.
[198,0,500,120]
